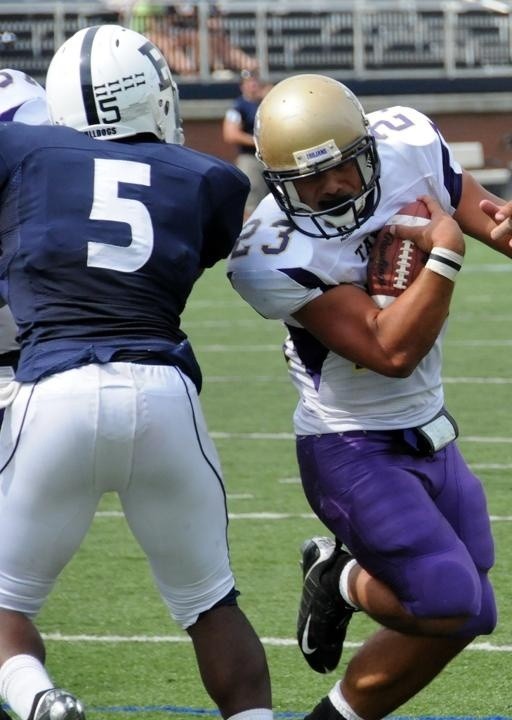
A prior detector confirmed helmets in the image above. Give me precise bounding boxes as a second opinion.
[250,72,383,241]
[44,24,187,148]
[1,68,48,128]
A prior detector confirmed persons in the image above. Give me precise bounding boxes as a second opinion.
[120,1,193,75]
[0,67,63,424]
[224,72,511,720]
[166,2,260,74]
[223,73,276,235]
[0,22,279,720]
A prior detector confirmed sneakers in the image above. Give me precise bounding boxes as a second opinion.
[295,535,357,675]
[27,686,86,719]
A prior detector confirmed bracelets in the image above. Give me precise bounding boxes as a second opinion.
[421,245,467,285]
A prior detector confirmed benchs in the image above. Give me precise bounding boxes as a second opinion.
[444,140,511,187]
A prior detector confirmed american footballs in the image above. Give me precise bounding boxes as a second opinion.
[365,201,431,311]
